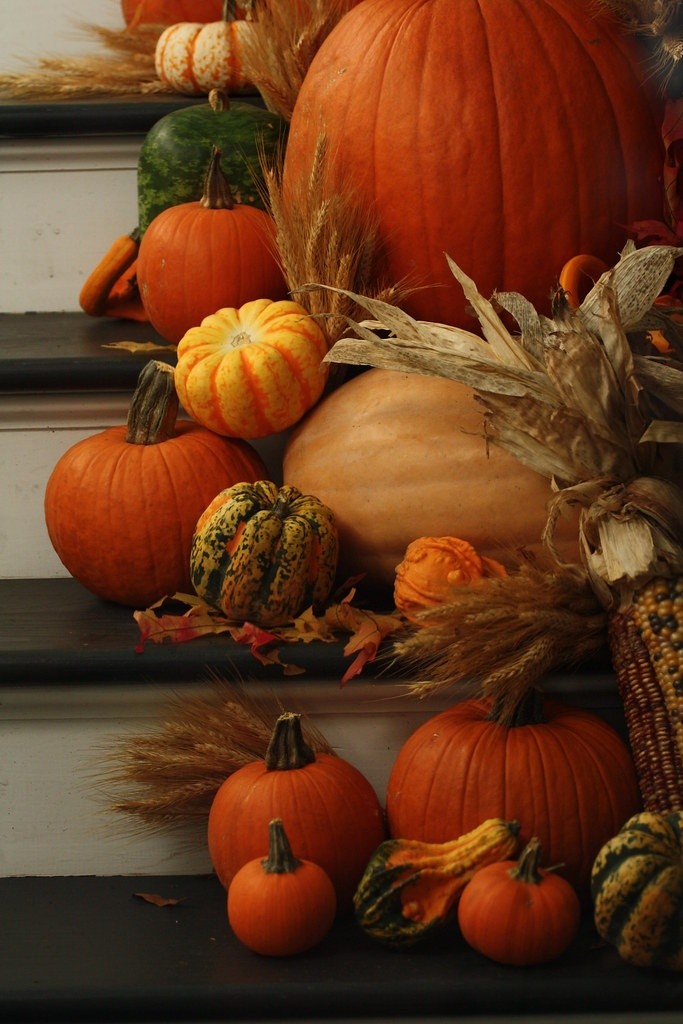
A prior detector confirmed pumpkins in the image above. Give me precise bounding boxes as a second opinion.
[45,0,683,971]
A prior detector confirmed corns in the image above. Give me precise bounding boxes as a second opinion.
[608,607,683,814]
[636,581,683,754]
